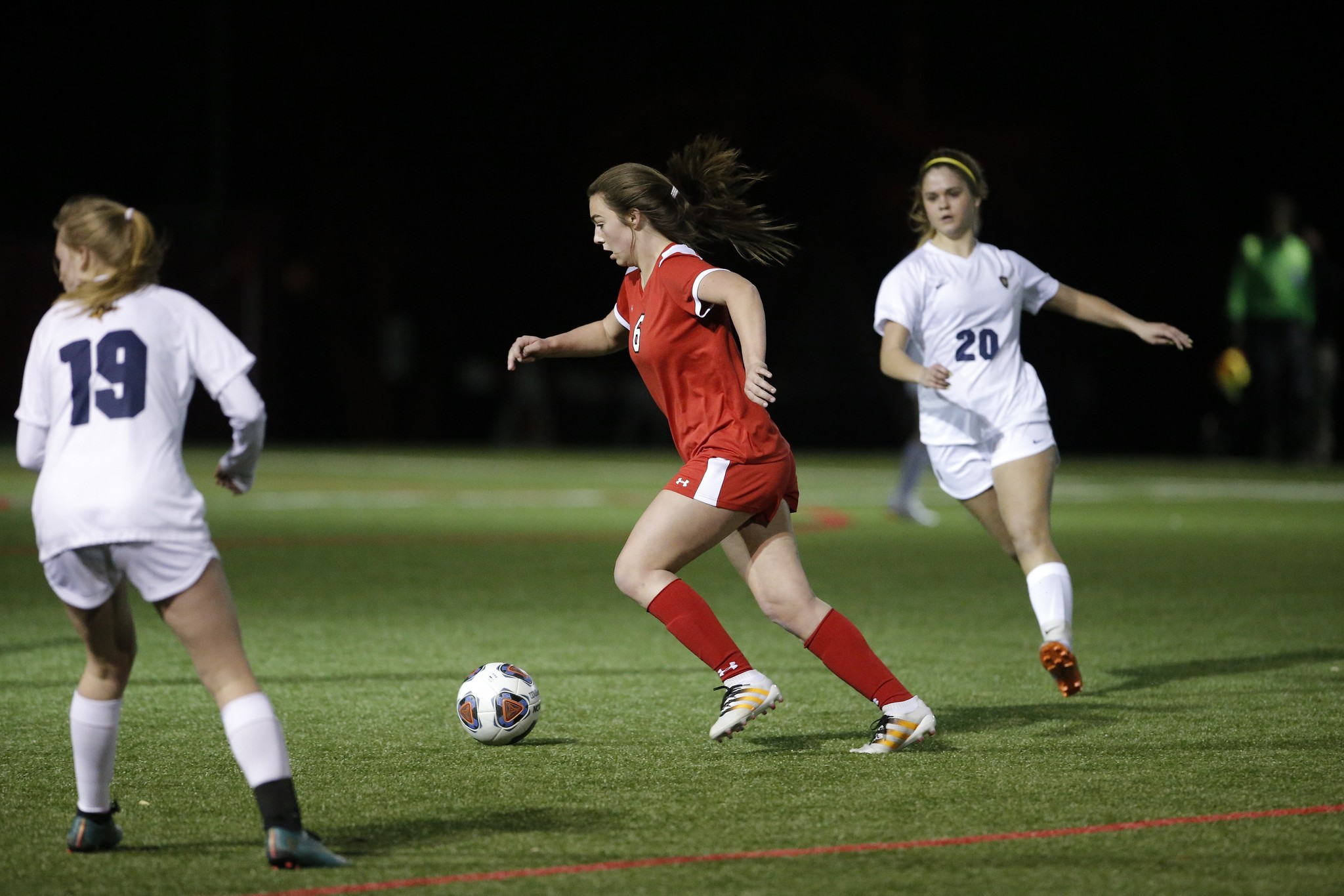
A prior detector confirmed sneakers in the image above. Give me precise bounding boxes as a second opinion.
[707,667,782,739]
[68,814,126,853]
[266,828,345,868]
[851,695,939,755]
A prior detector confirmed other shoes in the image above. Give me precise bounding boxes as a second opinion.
[1041,639,1083,698]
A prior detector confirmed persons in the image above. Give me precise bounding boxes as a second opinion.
[873,148,1192,698]
[1229,194,1313,460]
[508,132,935,755]
[893,381,938,525]
[14,196,355,870]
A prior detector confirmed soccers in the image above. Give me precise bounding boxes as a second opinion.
[457,662,541,746]
